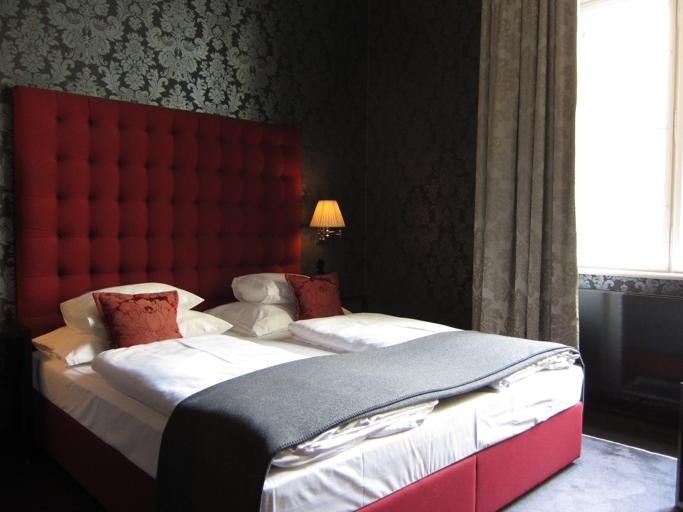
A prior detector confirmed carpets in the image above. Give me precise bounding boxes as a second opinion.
[495,432,682,512]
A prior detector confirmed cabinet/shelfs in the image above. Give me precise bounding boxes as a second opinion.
[0,331,34,497]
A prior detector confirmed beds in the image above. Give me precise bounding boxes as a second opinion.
[14,85,586,512]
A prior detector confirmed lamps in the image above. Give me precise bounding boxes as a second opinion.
[310,200,346,241]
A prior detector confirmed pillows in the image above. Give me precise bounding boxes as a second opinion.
[202,302,352,338]
[32,308,234,366]
[284,272,345,320]
[60,282,206,335]
[93,289,184,346]
[230,272,311,304]
